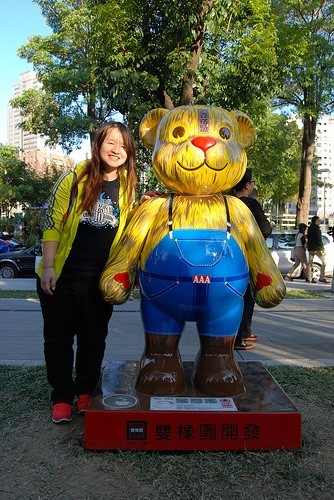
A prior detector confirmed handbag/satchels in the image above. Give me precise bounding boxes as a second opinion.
[291,248,295,262]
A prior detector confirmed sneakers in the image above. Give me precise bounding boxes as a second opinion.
[76,393,94,415]
[51,403,72,423]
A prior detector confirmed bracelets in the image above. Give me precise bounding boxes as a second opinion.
[43,266,54,268]
[138,201,141,206]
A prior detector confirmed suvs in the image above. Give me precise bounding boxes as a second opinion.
[265,229,334,281]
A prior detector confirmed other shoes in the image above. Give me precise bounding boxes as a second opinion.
[306,278,309,282]
[286,274,293,282]
[233,340,254,350]
[308,279,316,283]
[319,279,330,283]
[241,335,257,340]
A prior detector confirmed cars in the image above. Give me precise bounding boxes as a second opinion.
[0,243,42,278]
[0,238,26,253]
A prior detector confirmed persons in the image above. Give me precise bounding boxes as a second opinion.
[36,122,162,423]
[248,188,258,199]
[285,223,308,282]
[305,216,330,283]
[227,168,273,350]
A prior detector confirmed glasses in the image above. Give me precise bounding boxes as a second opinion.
[248,180,256,185]
